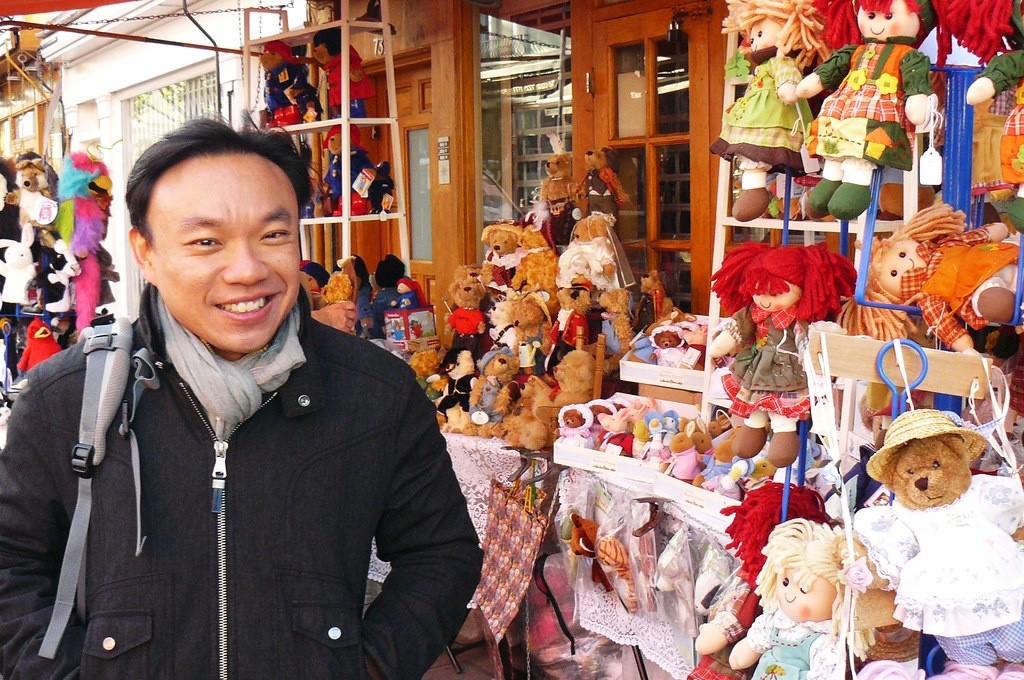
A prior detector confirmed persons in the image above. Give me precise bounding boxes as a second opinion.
[0,114,483,679]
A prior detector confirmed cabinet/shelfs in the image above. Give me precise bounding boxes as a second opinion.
[702,33,925,421]
[244,0,410,281]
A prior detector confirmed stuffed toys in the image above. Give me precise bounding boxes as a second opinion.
[0,1,1021,677]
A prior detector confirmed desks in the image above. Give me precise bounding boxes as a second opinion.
[553,470,742,680]
[366,433,554,677]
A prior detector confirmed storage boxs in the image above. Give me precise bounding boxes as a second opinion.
[537,312,787,529]
[383,306,439,352]
